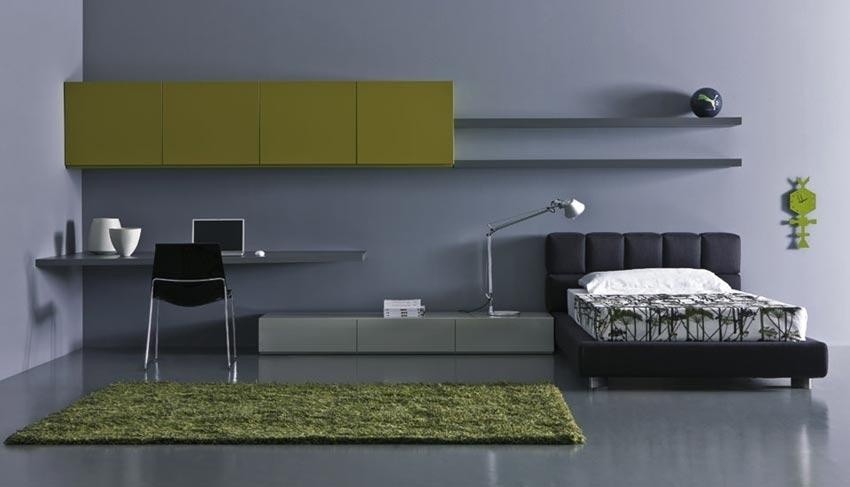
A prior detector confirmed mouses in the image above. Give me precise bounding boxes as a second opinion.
[255,250,266,257]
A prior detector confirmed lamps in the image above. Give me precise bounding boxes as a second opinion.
[472,196,586,316]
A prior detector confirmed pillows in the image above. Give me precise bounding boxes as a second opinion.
[577,266,733,297]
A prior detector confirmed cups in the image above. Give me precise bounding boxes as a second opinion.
[109,226,143,258]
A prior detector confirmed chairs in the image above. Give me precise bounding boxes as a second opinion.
[142,240,239,376]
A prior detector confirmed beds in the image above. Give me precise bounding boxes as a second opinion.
[545,231,829,390]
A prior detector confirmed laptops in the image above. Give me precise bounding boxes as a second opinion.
[191,217,246,258]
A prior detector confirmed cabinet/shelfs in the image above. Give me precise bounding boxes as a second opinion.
[61,78,453,171]
[451,115,744,170]
[255,311,556,357]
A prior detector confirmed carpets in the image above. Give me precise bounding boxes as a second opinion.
[3,375,587,447]
